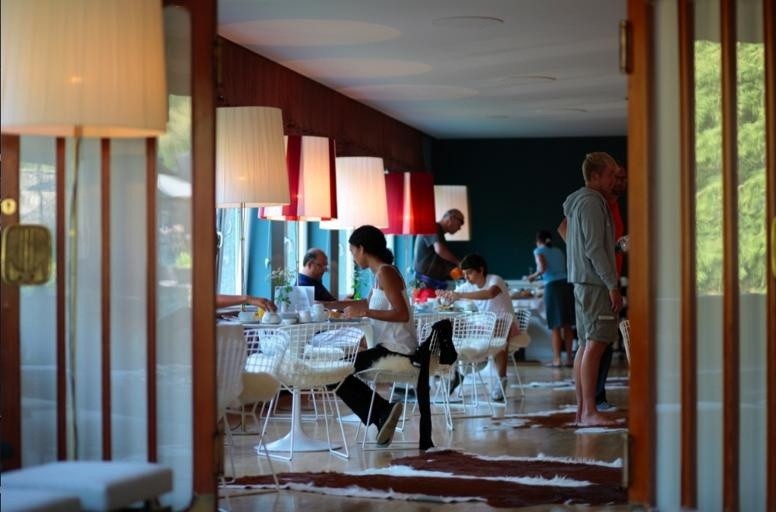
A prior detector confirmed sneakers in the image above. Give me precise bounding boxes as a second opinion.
[493,377,508,400]
[375,402,403,448]
[595,401,618,413]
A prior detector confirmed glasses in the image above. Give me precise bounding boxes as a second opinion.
[616,177,627,184]
[312,261,329,272]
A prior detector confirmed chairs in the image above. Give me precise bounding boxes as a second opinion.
[215,325,281,511]
[216,303,532,461]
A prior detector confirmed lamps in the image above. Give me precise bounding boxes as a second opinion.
[215,106,469,323]
[0,2,175,462]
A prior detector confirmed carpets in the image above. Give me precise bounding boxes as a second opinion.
[216,446,626,510]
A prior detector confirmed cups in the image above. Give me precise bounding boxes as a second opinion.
[299,303,331,323]
[239,311,256,321]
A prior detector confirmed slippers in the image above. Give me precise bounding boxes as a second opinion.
[543,360,562,368]
[563,361,573,367]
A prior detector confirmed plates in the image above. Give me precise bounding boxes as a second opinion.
[237,319,259,324]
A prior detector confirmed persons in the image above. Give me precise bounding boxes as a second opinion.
[215,232,278,312]
